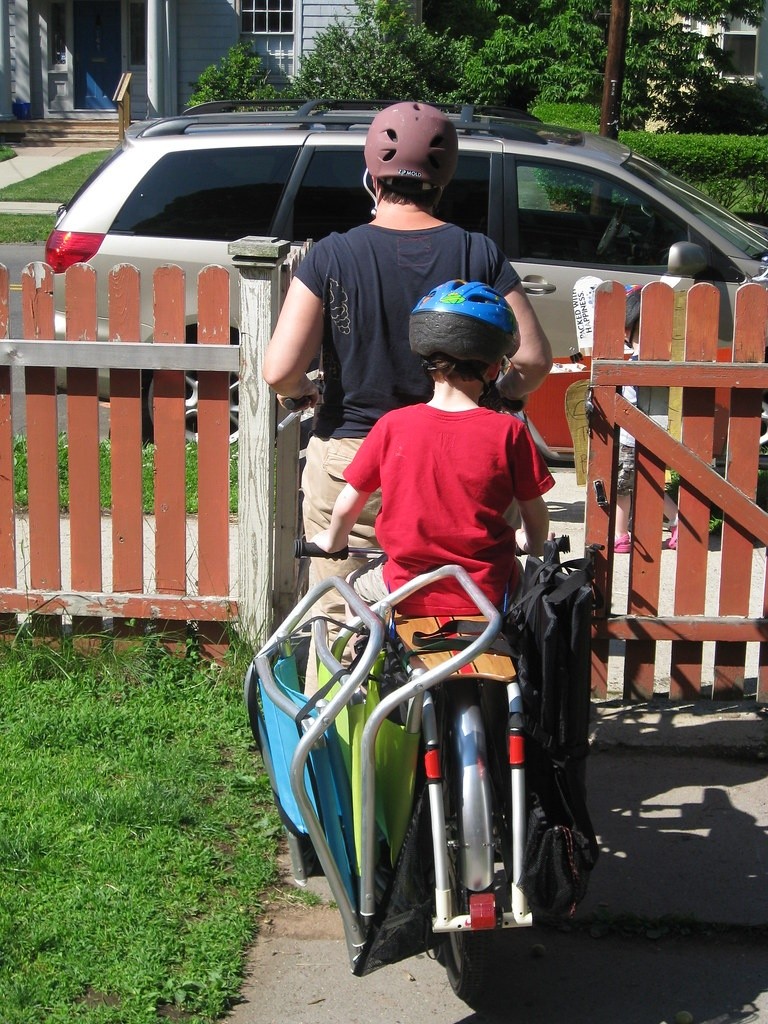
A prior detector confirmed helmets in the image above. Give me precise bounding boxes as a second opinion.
[409,279,521,366]
[364,101,459,186]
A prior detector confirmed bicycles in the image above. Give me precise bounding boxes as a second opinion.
[273,380,571,1001]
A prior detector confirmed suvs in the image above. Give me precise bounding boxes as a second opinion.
[37,106,768,435]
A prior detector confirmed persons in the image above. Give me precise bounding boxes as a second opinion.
[613,281,679,554]
[258,101,549,610]
[307,277,556,620]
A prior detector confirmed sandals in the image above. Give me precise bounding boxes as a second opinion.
[667,527,678,549]
[614,534,632,553]
